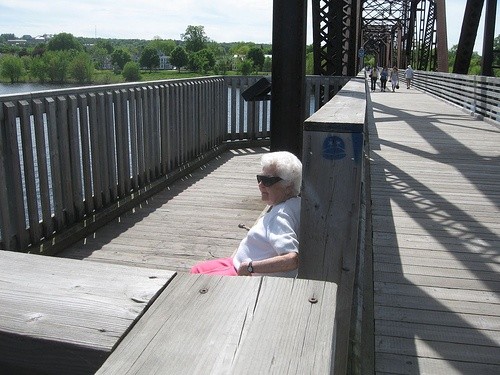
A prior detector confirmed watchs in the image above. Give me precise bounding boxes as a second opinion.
[247,261,255,273]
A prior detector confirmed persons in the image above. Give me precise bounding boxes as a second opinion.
[404,65,414,89]
[380,67,388,92]
[189,152,303,278]
[370,66,379,90]
[390,67,400,92]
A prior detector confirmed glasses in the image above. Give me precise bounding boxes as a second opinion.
[257,175,282,187]
